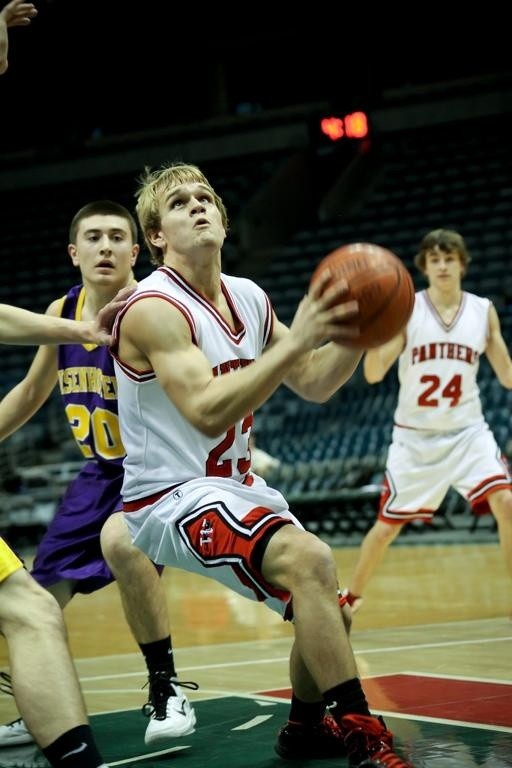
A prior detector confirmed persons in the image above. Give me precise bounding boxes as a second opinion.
[2,204,198,746]
[340,229,512,612]
[109,166,420,767]
[2,281,138,768]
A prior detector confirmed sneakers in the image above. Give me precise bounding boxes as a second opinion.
[0,714,35,746]
[141,674,199,746]
[272,713,407,768]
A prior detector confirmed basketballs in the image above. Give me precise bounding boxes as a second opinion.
[307,244,415,353]
